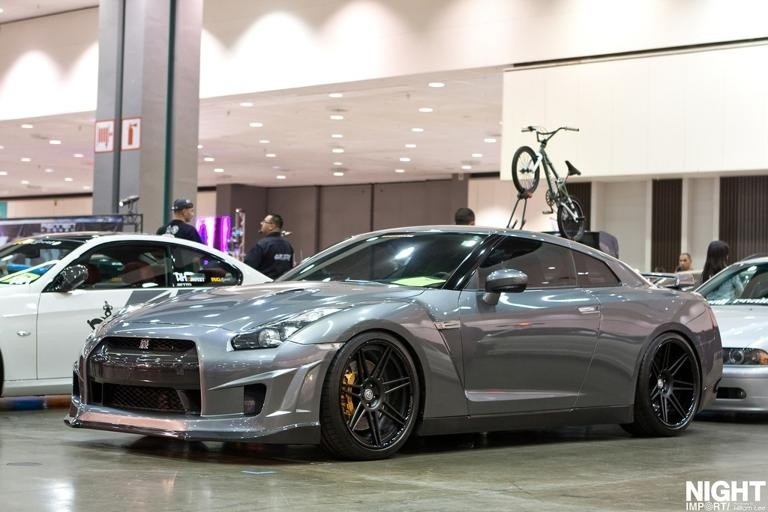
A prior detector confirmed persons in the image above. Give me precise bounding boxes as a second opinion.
[654,265,664,272]
[695,240,744,302]
[244,213,295,280]
[455,207,475,225]
[156,197,203,244]
[680,253,693,271]
[40,223,76,233]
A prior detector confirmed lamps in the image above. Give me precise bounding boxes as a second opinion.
[118,194,140,214]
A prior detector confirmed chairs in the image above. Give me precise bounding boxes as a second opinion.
[118,260,157,288]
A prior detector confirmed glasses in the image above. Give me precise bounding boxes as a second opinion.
[260,221,274,225]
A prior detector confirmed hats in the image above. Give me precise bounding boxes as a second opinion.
[172,197,192,210]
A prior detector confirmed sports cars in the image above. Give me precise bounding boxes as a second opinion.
[58,217,726,467]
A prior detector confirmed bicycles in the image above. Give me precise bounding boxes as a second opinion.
[507,124,589,243]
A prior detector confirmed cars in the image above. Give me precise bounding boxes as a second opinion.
[691,253,768,419]
[1,230,277,414]
